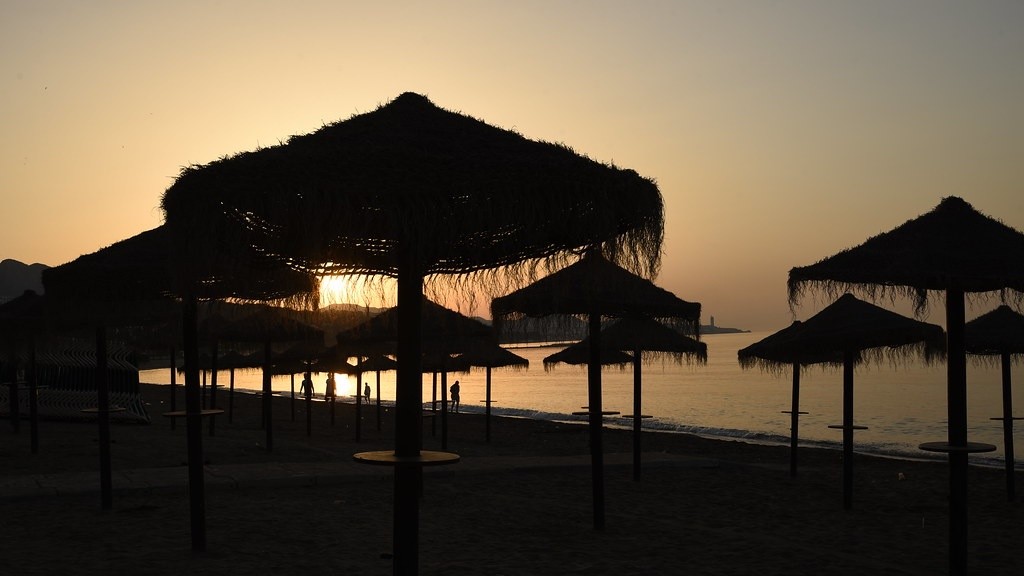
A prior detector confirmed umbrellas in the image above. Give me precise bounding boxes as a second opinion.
[2,92,1024,575]
